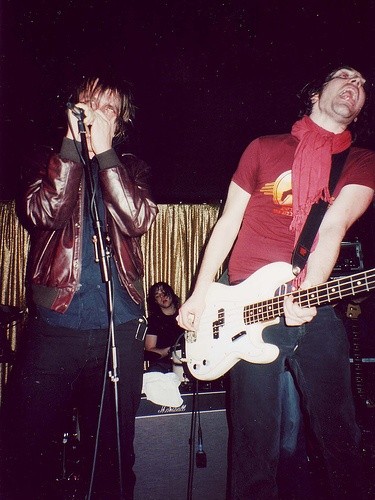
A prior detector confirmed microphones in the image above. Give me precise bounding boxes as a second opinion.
[196,426,207,468]
[66,102,91,128]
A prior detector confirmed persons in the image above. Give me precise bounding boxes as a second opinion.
[177,58,375,500]
[0,72,157,500]
[144,280,184,371]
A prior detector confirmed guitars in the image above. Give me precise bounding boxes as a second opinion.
[345,301,375,454]
[184,261,375,380]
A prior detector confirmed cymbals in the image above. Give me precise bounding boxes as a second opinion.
[144,350,162,361]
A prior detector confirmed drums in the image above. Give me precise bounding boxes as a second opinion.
[170,346,204,388]
